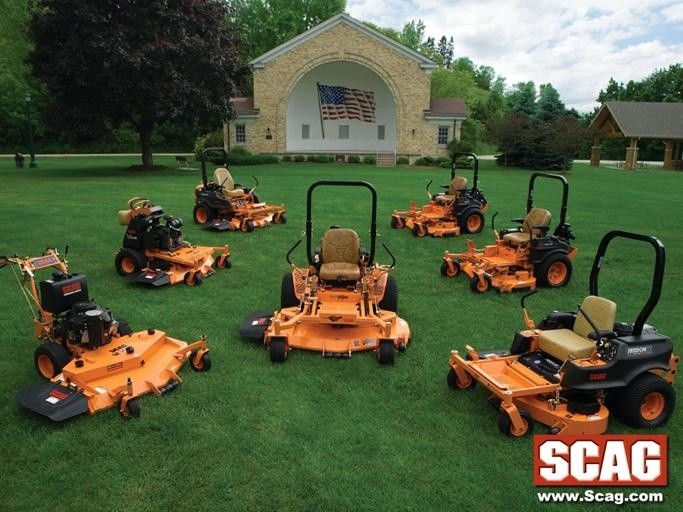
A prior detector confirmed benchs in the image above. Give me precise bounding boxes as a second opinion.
[174,156,191,169]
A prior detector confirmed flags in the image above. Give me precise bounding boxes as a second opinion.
[318,85,376,123]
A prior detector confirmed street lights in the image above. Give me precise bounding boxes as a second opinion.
[24,93,37,168]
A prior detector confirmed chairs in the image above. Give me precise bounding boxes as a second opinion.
[214,167,243,198]
[434,176,468,204]
[538,293,619,361]
[502,207,551,248]
[319,229,359,280]
